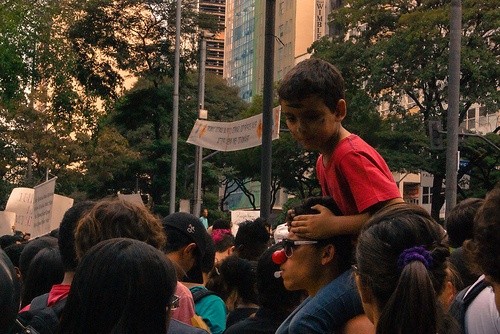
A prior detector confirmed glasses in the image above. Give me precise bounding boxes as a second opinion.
[282,238,328,258]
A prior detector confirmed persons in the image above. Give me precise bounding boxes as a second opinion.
[0,181,500,334]
[277,58,406,334]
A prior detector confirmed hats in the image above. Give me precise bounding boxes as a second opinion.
[160,211,208,259]
[274,224,290,244]
[18,236,58,286]
